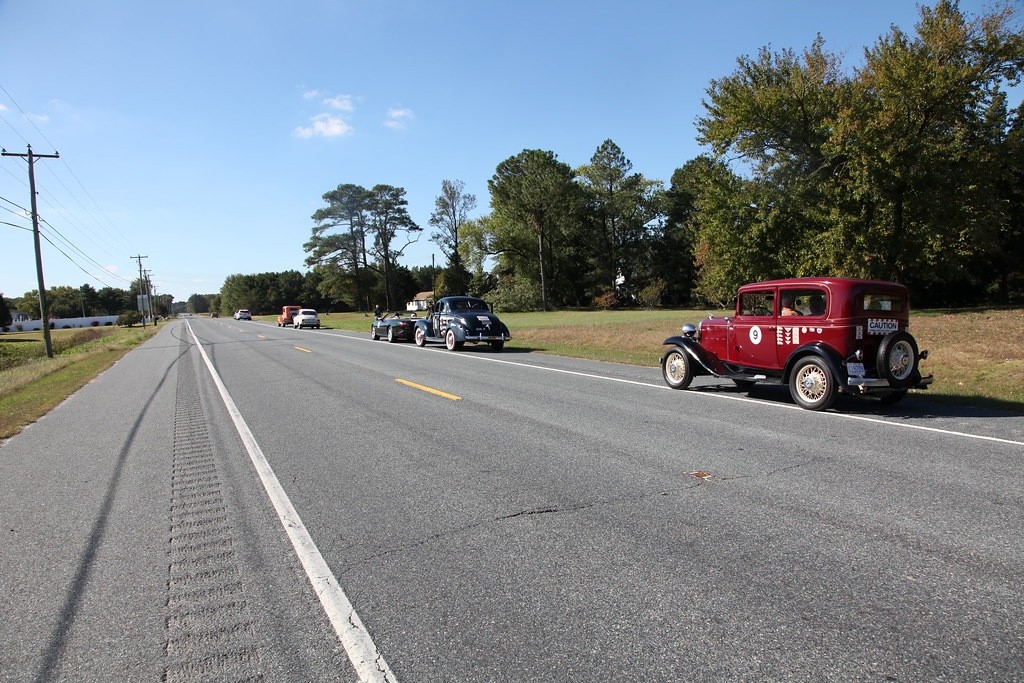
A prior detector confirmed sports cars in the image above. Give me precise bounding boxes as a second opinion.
[371,309,424,343]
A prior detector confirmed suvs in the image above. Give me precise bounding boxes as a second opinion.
[658,276,934,412]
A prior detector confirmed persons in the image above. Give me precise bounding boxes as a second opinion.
[410,312,416,317]
[430,303,433,319]
[781,294,802,315]
[809,294,824,315]
[425,301,432,318]
[390,312,399,318]
[867,299,882,309]
[373,304,382,320]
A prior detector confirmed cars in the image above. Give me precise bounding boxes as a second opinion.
[211,312,218,318]
[277,306,302,327]
[234,309,252,321]
[413,295,512,353]
[293,308,320,330]
